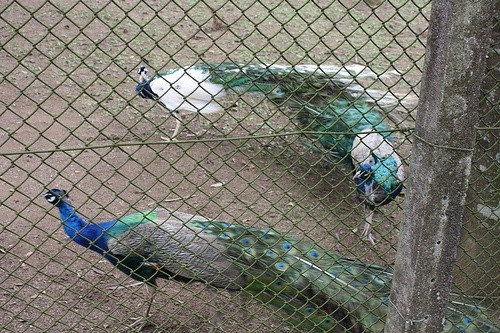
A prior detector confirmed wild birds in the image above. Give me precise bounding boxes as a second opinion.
[266,76,404,251]
[42,189,499,333]
[135,64,421,144]
